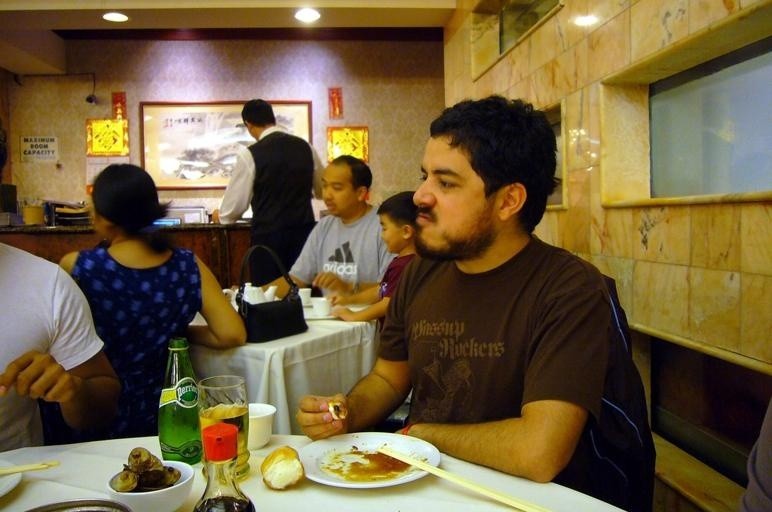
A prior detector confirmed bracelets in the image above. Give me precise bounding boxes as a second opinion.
[401,423,413,435]
[352,281,361,294]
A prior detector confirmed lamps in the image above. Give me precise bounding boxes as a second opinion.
[10,70,98,105]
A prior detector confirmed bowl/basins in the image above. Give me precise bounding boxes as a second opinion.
[246,402,278,450]
[106,460,196,511]
[23,497,132,512]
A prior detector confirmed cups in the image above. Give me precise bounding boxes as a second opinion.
[298,286,312,307]
[197,373,252,483]
[309,294,330,316]
[22,204,47,227]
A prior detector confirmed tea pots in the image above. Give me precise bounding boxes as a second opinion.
[222,286,240,313]
[248,286,279,304]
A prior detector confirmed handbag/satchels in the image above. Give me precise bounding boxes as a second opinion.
[235,243,308,344]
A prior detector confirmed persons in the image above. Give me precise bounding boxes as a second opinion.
[743,398,772,512]
[211,98,324,288]
[327,190,419,339]
[56,164,246,439]
[0,243,121,457]
[224,155,400,297]
[296,93,655,512]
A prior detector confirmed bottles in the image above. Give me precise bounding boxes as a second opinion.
[156,336,204,464]
[243,282,253,299]
[193,424,257,511]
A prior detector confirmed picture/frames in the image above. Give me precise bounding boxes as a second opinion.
[137,98,314,191]
[84,115,131,159]
[325,124,370,164]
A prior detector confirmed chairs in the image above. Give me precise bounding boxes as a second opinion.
[353,273,655,512]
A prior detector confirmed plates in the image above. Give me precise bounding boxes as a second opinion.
[1,459,22,500]
[303,312,339,320]
[299,431,443,489]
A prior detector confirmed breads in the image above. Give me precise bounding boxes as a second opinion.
[261,446,305,490]
